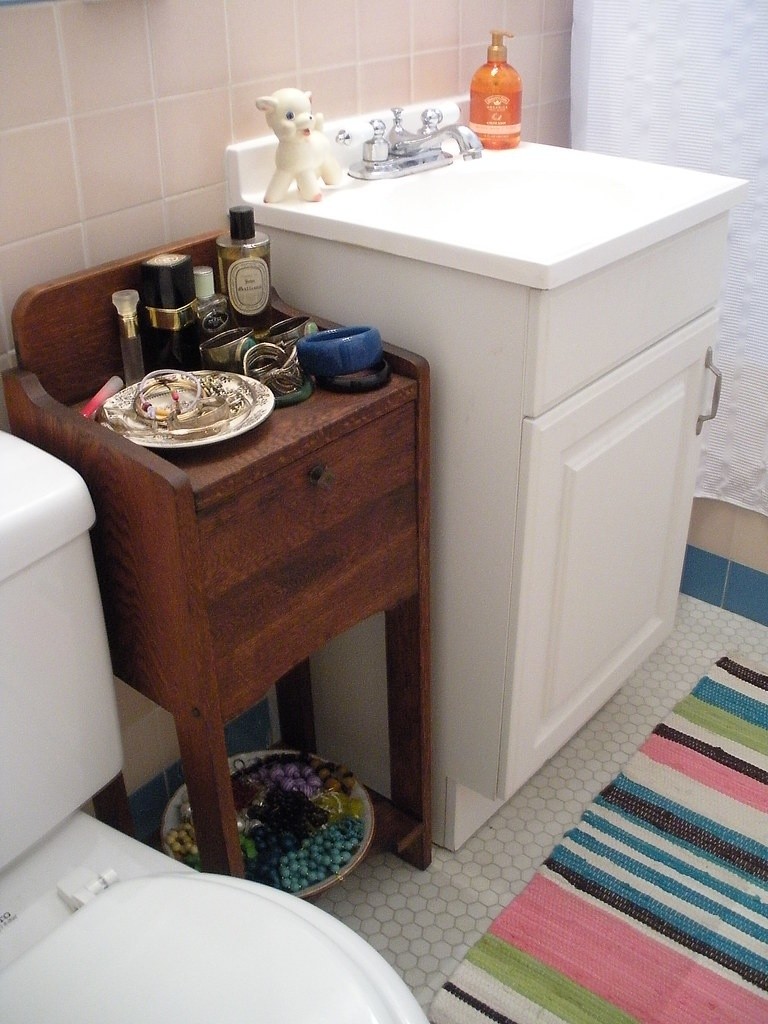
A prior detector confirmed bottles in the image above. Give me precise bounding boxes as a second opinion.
[112,290,146,388]
[215,205,271,328]
[192,265,230,338]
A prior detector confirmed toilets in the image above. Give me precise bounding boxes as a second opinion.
[1,429,432,1024]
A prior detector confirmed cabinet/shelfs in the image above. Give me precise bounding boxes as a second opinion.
[0,230,433,882]
[254,210,730,853]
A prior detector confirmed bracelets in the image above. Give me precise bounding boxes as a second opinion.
[99,316,390,439]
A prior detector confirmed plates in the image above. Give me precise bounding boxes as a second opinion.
[97,370,275,447]
[160,748,376,897]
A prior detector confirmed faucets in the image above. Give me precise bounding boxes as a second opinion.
[387,108,484,161]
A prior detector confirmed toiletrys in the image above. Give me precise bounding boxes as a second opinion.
[468,30,521,149]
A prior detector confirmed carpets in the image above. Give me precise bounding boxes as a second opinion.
[429,657,768,1022]
